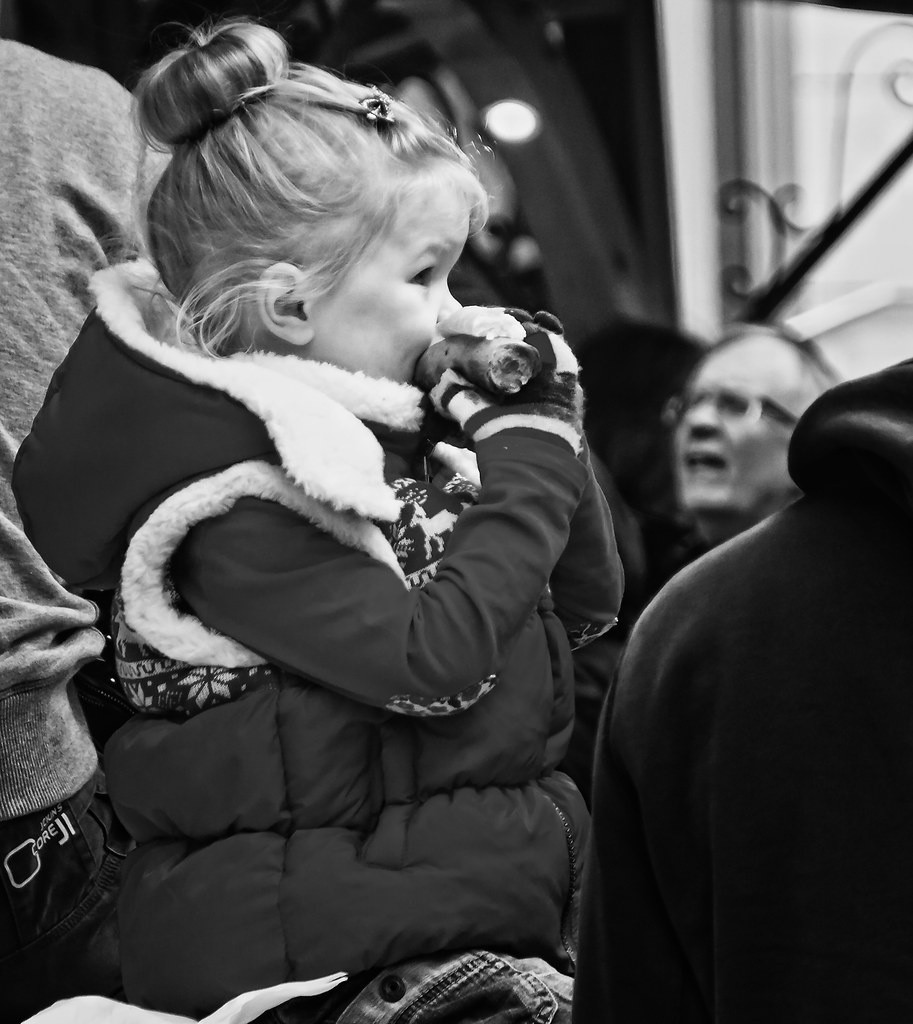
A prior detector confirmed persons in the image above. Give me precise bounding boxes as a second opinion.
[0,32,183,1024]
[562,319,840,815]
[13,19,625,1024]
[571,355,912,1024]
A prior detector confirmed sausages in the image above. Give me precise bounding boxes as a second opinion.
[413,334,540,393]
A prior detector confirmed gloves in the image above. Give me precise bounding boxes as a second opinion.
[486,304,563,338]
[442,325,587,454]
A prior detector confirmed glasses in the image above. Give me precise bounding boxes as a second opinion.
[665,388,799,431]
